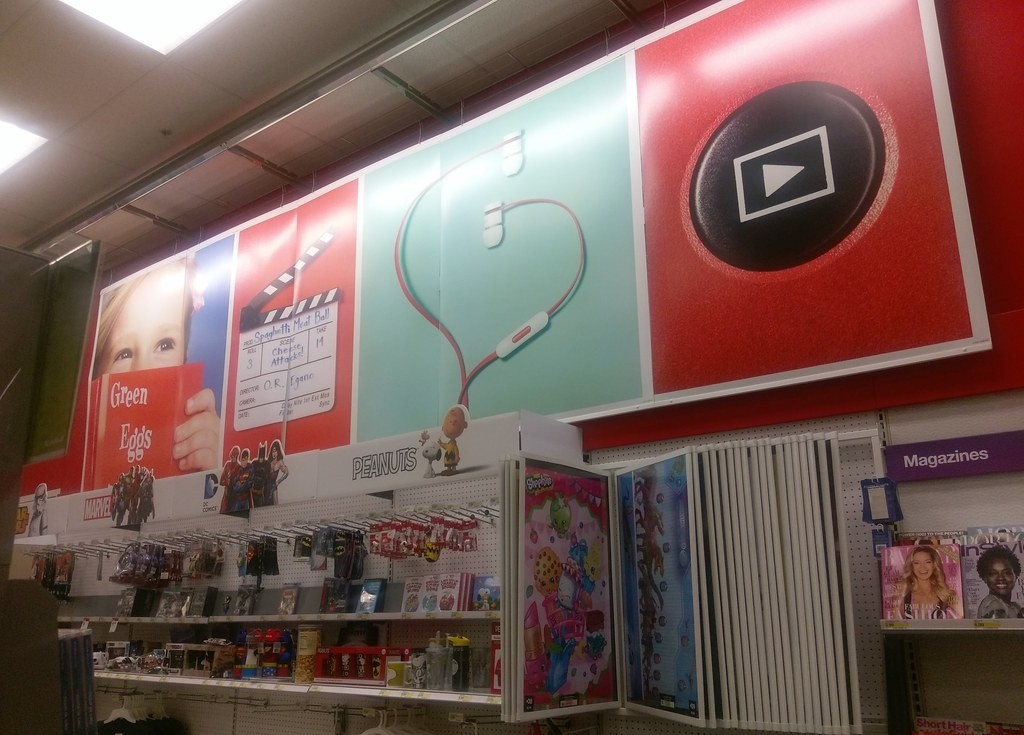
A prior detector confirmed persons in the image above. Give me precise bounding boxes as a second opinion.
[977,547,1024,619]
[92,255,220,471]
[891,546,963,619]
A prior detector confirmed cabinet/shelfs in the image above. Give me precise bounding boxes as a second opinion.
[52,610,503,706]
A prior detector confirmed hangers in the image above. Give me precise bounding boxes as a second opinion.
[96,690,188,735]
[356,704,437,735]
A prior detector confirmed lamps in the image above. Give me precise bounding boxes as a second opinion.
[860,477,898,527]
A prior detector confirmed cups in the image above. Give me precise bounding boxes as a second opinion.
[384,661,411,690]
[327,652,403,681]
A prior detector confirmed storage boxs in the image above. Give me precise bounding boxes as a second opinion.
[114,585,219,617]
[355,573,474,611]
[313,643,417,687]
[105,640,144,669]
[160,643,223,678]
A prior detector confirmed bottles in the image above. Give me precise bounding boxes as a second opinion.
[409,631,470,691]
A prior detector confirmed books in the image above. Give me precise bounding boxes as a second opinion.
[86,363,203,491]
